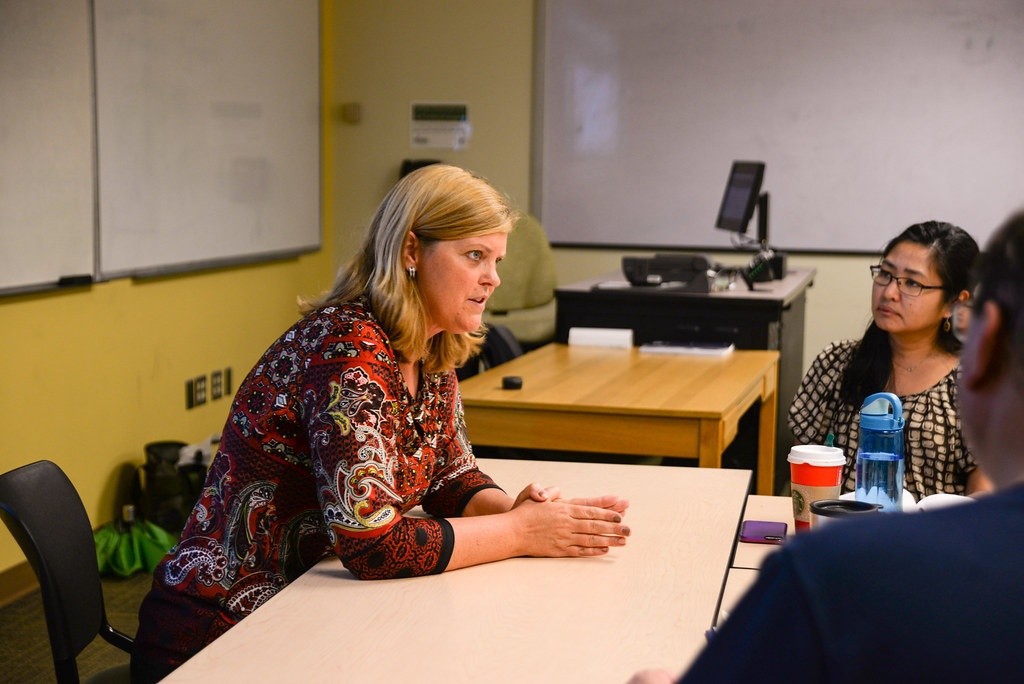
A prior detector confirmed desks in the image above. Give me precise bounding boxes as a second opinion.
[553,263,820,492]
[480,212,557,342]
[158,458,752,684]
[457,343,779,495]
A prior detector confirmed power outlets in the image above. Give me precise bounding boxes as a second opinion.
[211,367,231,402]
[187,374,207,408]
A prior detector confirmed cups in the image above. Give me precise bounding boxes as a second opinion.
[809,499,882,531]
[787,444,847,534]
[855,392,906,512]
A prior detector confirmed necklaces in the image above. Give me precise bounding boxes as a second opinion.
[892,345,935,372]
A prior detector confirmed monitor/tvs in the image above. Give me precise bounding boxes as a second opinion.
[715,159,768,247]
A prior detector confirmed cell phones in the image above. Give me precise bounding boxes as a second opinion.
[740,520,787,545]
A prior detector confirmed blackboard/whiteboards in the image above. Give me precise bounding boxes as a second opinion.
[531,0,1023,258]
[0,1,323,299]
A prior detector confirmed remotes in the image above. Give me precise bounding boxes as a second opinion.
[502,376,522,389]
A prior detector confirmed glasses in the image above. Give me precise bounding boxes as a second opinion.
[870,265,943,297]
[953,301,1016,341]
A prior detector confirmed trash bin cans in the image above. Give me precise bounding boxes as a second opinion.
[143,441,203,531]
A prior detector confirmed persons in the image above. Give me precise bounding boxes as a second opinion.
[630,203,1024,684]
[790,222,997,499]
[135,164,628,684]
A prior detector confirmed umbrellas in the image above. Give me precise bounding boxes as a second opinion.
[90,502,176,576]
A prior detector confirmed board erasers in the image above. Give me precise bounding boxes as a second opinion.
[58,274,92,287]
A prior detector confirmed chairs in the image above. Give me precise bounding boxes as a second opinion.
[0,460,135,684]
